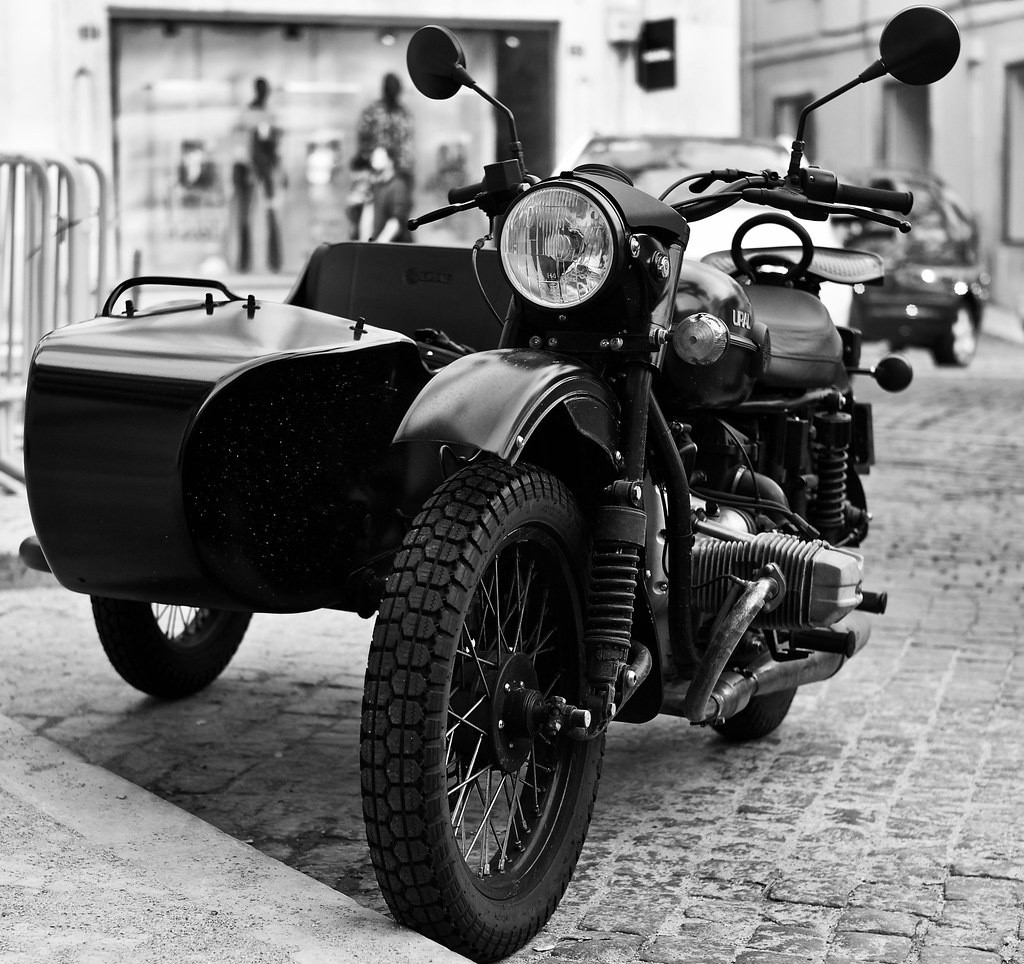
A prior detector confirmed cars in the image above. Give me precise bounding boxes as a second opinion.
[567,133,858,370]
[833,169,994,368]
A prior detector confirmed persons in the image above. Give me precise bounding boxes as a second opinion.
[175,141,223,210]
[350,143,414,244]
[228,77,286,274]
[357,73,415,185]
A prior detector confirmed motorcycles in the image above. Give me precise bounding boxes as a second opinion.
[20,6,960,964]
